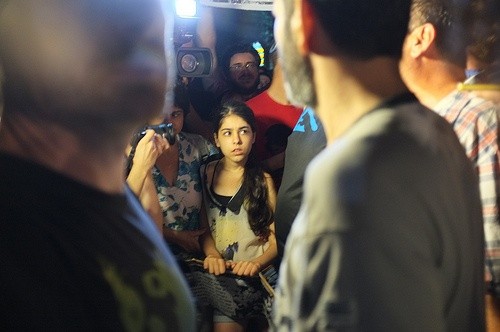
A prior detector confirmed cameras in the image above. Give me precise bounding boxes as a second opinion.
[133,123,175,148]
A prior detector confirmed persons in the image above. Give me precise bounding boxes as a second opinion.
[399,0,500,332]
[272,0,486,332]
[196,103,277,332]
[275,104,330,269]
[0,0,195,332]
[130,41,324,249]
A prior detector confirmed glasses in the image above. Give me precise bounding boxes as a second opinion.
[229,64,257,72]
